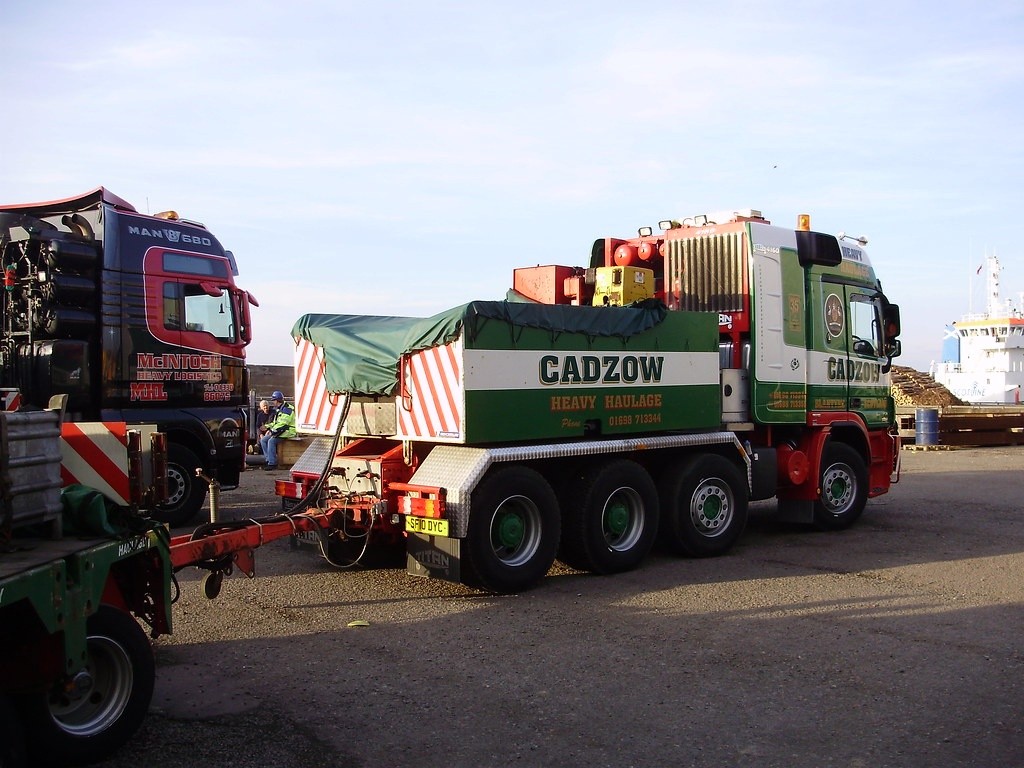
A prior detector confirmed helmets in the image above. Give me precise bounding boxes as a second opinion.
[270,390,283,402]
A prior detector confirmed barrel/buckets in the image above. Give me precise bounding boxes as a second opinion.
[915,410,938,446]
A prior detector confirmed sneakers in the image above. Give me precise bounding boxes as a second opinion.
[264,463,278,472]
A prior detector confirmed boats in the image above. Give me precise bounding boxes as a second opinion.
[934,255,1022,402]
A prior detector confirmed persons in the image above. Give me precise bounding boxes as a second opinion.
[260,390,296,470]
[256,400,277,439]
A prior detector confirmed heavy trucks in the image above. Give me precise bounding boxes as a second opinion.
[1,187,260,530]
[1,209,903,768]
